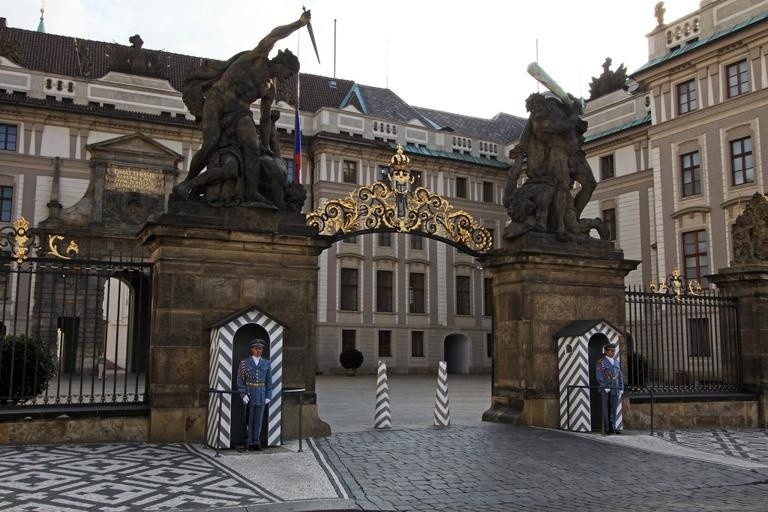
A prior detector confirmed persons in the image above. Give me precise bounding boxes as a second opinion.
[500,88,614,242]
[162,10,315,213]
[168,107,308,216]
[594,343,626,436]
[235,337,275,449]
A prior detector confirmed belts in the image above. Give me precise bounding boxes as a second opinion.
[246,382,265,386]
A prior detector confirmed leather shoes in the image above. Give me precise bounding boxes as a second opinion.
[244,444,263,453]
[604,429,622,435]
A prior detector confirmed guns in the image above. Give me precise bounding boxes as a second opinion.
[608,383,615,434]
[243,393,251,451]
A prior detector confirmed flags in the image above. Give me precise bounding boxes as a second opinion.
[292,108,306,185]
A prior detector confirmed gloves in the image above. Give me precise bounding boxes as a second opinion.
[619,390,624,394]
[604,388,610,393]
[265,398,270,404]
[243,395,250,404]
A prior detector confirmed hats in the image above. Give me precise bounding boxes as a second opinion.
[604,344,617,351]
[249,340,266,348]
